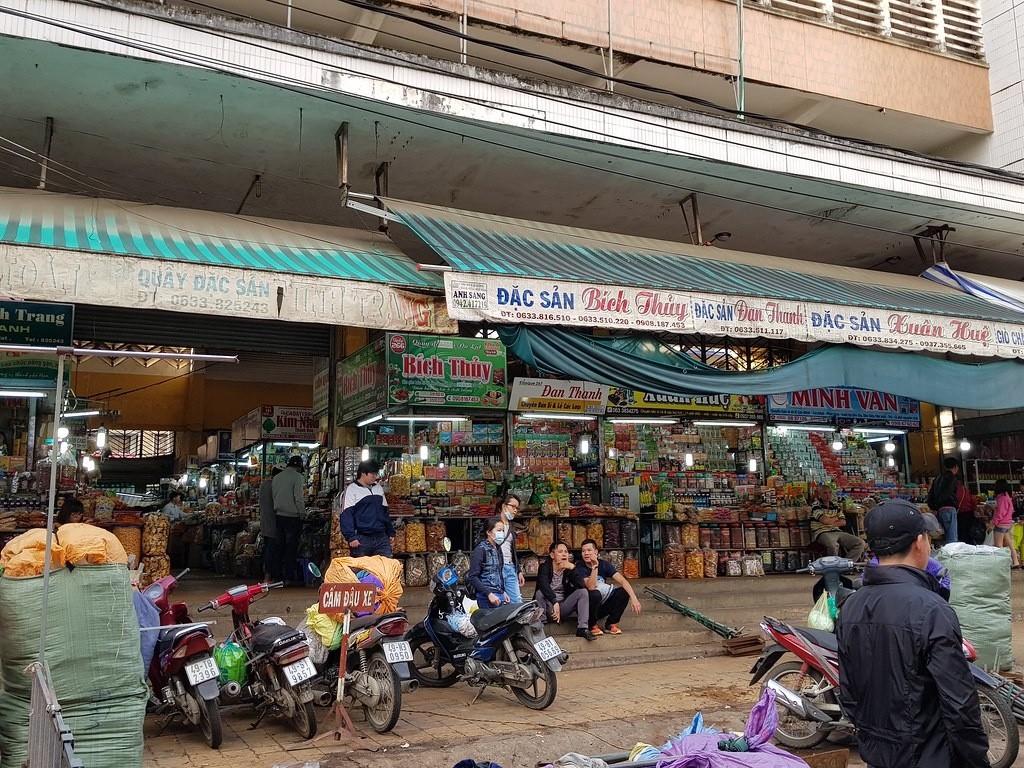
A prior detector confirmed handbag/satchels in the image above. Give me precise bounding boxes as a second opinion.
[983,528,994,546]
[214,637,249,686]
[807,589,834,633]
[463,545,486,600]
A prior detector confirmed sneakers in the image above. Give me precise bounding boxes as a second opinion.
[590,625,604,636]
[604,624,622,634]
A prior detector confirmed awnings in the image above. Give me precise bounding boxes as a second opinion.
[342,188,1024,362]
[1,188,461,335]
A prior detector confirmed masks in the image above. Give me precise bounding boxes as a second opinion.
[504,509,514,520]
[490,531,504,545]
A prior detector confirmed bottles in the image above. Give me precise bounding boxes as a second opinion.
[1012,491,1024,509]
[701,522,810,547]
[92,481,135,495]
[0,498,41,513]
[571,492,592,505]
[575,468,599,488]
[410,497,435,517]
[761,488,777,509]
[837,483,931,503]
[609,491,629,510]
[658,474,757,489]
[672,494,710,508]
[419,486,449,506]
[710,493,737,505]
[444,446,500,467]
[1013,516,1019,523]
[146,484,160,492]
[976,501,986,516]
[836,455,858,476]
[777,495,794,507]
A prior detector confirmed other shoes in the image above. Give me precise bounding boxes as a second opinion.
[1011,565,1021,570]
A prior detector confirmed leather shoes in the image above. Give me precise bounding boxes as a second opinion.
[576,628,597,640]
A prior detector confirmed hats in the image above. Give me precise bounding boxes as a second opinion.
[284,456,303,468]
[863,499,940,556]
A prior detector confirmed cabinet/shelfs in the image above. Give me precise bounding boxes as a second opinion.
[643,520,829,577]
[182,441,317,504]
[360,418,905,505]
[389,516,641,579]
[962,450,1024,496]
[843,513,875,561]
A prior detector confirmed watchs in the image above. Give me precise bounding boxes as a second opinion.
[592,565,599,569]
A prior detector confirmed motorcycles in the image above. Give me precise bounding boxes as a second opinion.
[405,537,570,710]
[748,616,1020,768]
[141,569,241,749]
[794,556,866,634]
[306,561,420,733]
[197,581,333,740]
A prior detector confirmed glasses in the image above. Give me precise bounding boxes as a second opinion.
[505,503,520,511]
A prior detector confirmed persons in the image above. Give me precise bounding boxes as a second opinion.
[833,501,992,768]
[492,494,525,604]
[466,517,511,608]
[257,462,286,581]
[340,458,395,558]
[810,484,865,576]
[530,542,597,640]
[1019,479,1024,494]
[571,539,642,634]
[271,456,307,585]
[990,479,1019,570]
[924,456,976,545]
[162,492,195,522]
[52,497,84,528]
